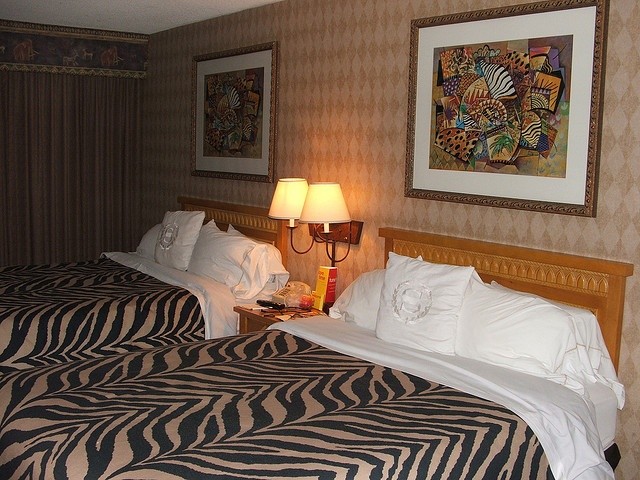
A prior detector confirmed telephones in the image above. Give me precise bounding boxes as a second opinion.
[273,281,312,305]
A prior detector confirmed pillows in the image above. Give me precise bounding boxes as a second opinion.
[375,251,475,357]
[154,210,205,270]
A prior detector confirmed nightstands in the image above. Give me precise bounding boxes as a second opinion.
[233,304,328,334]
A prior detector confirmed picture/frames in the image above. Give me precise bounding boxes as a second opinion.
[190,41,280,183]
[404,0,610,218]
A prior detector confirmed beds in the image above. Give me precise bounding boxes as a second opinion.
[0,227,634,479]
[0,195,288,370]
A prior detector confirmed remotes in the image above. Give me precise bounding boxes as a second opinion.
[256,299,285,311]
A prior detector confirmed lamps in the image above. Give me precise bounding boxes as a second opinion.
[297,182,351,234]
[267,176,308,229]
